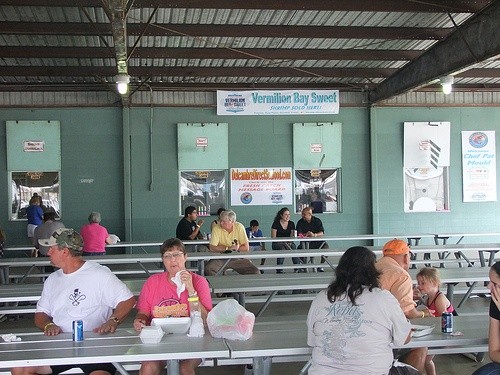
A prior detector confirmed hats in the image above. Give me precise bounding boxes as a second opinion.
[38,228,84,251]
[382,239,416,256]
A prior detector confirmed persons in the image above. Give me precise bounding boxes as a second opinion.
[11,228,136,375]
[414,267,459,375]
[33,213,66,279]
[133,238,213,375]
[211,208,226,231]
[472,261,500,375]
[176,206,209,252]
[271,207,301,274]
[374,238,436,374]
[79,211,113,256]
[306,246,421,375]
[0,229,7,259]
[296,207,329,272]
[25,192,45,258]
[245,220,267,274]
[204,209,261,298]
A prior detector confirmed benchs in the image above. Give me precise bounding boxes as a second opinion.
[1,309,42,317]
[216,287,490,308]
[3,258,496,276]
[122,345,491,372]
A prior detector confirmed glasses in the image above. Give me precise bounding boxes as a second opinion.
[162,252,184,260]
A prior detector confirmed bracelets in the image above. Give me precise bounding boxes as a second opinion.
[421,310,425,319]
[44,322,54,330]
[188,291,197,297]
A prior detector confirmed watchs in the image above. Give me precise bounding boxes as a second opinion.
[109,316,121,325]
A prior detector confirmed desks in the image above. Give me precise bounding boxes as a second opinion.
[0,233,500,375]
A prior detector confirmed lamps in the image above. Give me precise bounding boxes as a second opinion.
[114,74,130,94]
[440,77,455,94]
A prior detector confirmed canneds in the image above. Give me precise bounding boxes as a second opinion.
[72,320,83,342]
[248,231,252,238]
[441,311,454,333]
[207,233,211,241]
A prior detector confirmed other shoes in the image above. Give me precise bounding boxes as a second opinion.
[317,268,324,272]
